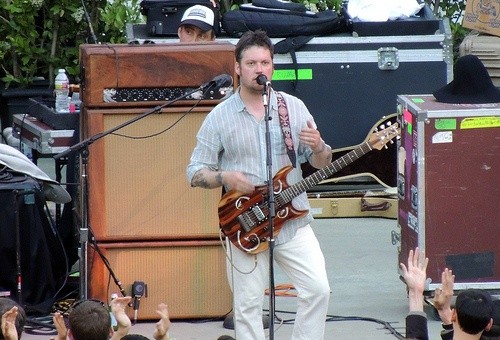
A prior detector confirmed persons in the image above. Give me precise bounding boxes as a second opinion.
[0,296,171,340]
[186,30,332,340]
[401,246,493,340]
[178,4,216,42]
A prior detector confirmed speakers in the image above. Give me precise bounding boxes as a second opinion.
[88,239,235,321]
[80,106,228,241]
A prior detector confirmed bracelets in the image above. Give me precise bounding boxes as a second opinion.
[216,172,223,185]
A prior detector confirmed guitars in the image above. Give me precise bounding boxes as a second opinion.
[218,121,401,255]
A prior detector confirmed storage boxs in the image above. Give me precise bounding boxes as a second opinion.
[397,95,500,290]
[11,113,75,155]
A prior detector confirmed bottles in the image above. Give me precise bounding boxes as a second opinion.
[55,68,70,113]
[108,293,119,331]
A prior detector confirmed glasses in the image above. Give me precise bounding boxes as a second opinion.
[71,299,104,309]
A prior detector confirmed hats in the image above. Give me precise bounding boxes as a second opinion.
[178,4,217,32]
[433,55,500,104]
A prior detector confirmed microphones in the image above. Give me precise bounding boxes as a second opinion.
[209,73,234,87]
[256,73,272,86]
[129,281,144,321]
